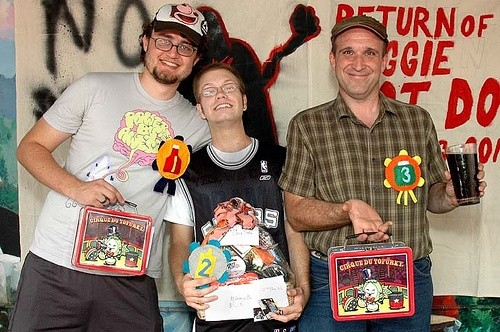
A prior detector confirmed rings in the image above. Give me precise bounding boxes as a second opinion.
[100,197,107,203]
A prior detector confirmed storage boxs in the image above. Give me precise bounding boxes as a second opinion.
[328,231,415,321]
[71,201,153,277]
[203,275,290,321]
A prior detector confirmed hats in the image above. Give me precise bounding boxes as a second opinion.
[331,14,388,41]
[153,2,209,52]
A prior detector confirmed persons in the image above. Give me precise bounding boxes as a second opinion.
[277,15,488,332]
[162,62,311,332]
[10,4,215,332]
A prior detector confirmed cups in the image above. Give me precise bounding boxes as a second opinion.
[444,142,481,207]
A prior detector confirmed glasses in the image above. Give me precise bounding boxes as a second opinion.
[202,83,239,98]
[151,36,196,57]
